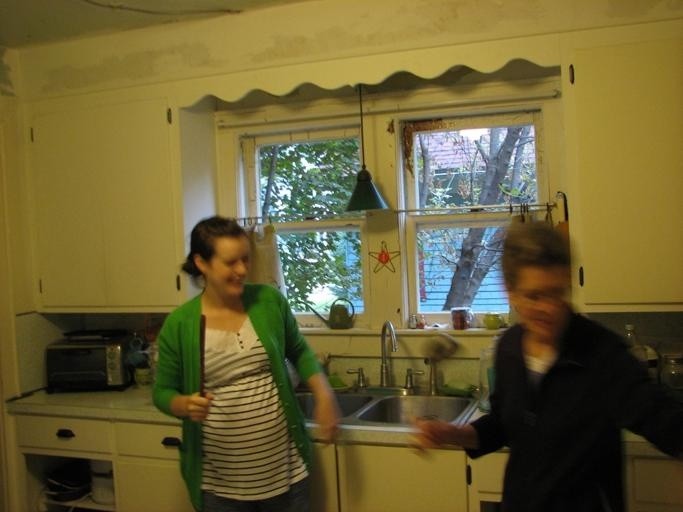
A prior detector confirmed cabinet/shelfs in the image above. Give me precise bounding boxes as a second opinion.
[560,26,681,315]
[115,418,193,510]
[306,424,467,510]
[23,96,218,317]
[18,414,114,511]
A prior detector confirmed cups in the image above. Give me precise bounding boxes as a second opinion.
[134,367,152,390]
[451,307,469,330]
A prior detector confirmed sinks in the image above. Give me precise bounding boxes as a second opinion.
[293,388,373,425]
[356,393,479,431]
[378,320,398,388]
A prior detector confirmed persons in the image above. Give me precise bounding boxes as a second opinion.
[414,222,683,512]
[151,216,340,512]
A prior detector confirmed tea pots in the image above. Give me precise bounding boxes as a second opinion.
[297,298,355,329]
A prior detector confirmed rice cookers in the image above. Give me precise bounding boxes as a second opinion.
[91,474,116,504]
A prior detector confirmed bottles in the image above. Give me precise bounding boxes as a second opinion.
[482,312,504,330]
[660,352,683,390]
[622,324,636,347]
[478,339,497,412]
[409,314,425,329]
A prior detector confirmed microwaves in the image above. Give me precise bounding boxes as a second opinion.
[43,328,132,392]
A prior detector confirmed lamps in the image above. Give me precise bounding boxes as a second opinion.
[345,82,389,215]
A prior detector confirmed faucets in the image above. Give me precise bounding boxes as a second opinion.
[424,356,439,396]
